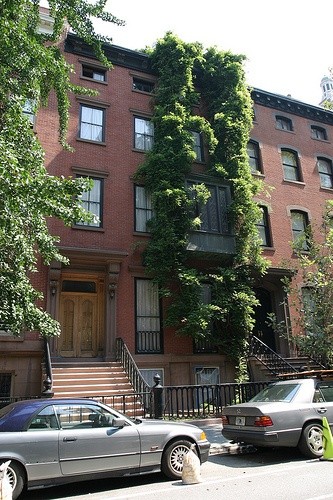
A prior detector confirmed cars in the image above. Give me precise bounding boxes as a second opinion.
[0,397,211,500]
[221,379,333,458]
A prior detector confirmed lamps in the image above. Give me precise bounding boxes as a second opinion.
[110,283,116,297]
[50,280,58,297]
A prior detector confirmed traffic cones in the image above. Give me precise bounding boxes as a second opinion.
[319,417,333,461]
